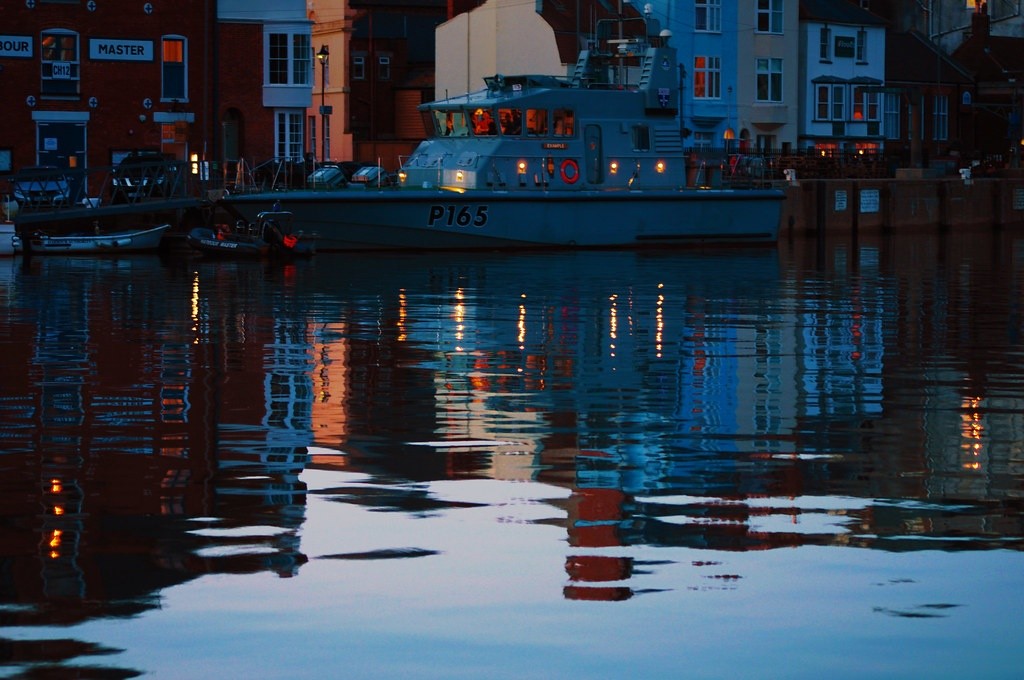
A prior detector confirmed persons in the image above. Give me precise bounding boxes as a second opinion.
[445,111,574,136]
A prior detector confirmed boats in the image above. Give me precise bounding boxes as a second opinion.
[215,1,789,258]
[186,204,321,257]
[24,214,173,254]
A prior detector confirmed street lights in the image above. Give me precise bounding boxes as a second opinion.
[316,43,331,170]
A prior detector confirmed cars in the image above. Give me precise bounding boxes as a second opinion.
[6,164,77,211]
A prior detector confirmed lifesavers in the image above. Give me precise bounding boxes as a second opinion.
[560,159,580,184]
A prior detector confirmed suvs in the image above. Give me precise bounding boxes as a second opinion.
[108,150,190,205]
